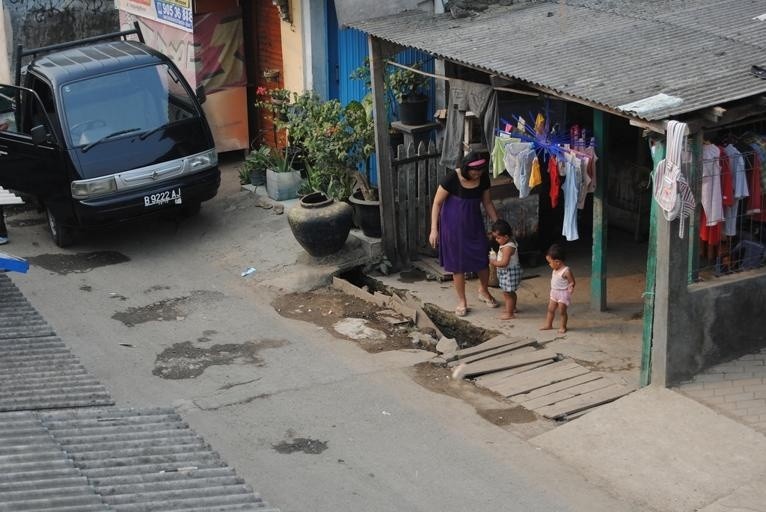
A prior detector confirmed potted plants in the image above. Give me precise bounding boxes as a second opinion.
[238,144,271,186]
[253,146,303,202]
[286,90,381,238]
[388,59,430,126]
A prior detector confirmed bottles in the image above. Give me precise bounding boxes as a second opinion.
[488,247,497,273]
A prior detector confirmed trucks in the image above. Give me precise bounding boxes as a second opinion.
[0,18,219,248]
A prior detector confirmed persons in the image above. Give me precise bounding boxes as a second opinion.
[428,152,500,317]
[540,244,575,333]
[0,110,15,245]
[490,219,521,320]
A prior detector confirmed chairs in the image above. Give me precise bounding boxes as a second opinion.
[712,240,765,276]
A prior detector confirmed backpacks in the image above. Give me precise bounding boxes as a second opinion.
[653,120,696,239]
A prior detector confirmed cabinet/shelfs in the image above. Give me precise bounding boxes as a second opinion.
[119,1,251,154]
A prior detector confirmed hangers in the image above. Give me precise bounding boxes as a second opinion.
[704,124,760,148]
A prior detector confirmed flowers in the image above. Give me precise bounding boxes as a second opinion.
[255,85,289,100]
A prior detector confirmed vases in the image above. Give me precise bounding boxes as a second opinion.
[271,98,283,105]
[286,190,354,256]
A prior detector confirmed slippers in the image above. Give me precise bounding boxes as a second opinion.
[455,307,467,317]
[478,295,499,308]
